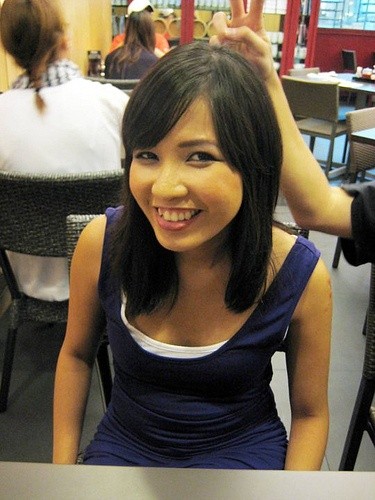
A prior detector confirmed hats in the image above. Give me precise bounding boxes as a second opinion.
[126,0,154,15]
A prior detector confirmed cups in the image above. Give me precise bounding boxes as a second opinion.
[88,50,101,76]
[362,69,371,80]
[356,67,362,77]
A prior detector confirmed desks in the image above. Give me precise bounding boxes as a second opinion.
[0,460,375,500]
[292,71,375,111]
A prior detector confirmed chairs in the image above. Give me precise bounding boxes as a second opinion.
[0,75,375,471]
[341,49,357,73]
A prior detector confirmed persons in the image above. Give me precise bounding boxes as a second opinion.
[0,0,130,305]
[205,1,375,350]
[102,0,171,79]
[52,43,331,473]
